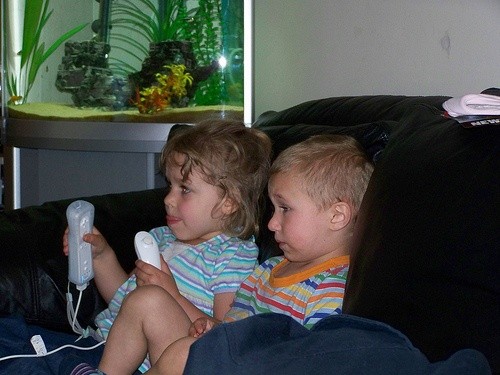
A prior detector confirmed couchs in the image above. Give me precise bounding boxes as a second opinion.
[0,91,500,374]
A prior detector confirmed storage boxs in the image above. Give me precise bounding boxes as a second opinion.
[0,0,255,134]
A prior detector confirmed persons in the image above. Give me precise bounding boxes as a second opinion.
[0,112,278,375]
[55,132,385,375]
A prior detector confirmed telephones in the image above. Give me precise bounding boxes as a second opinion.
[135,230,162,271]
